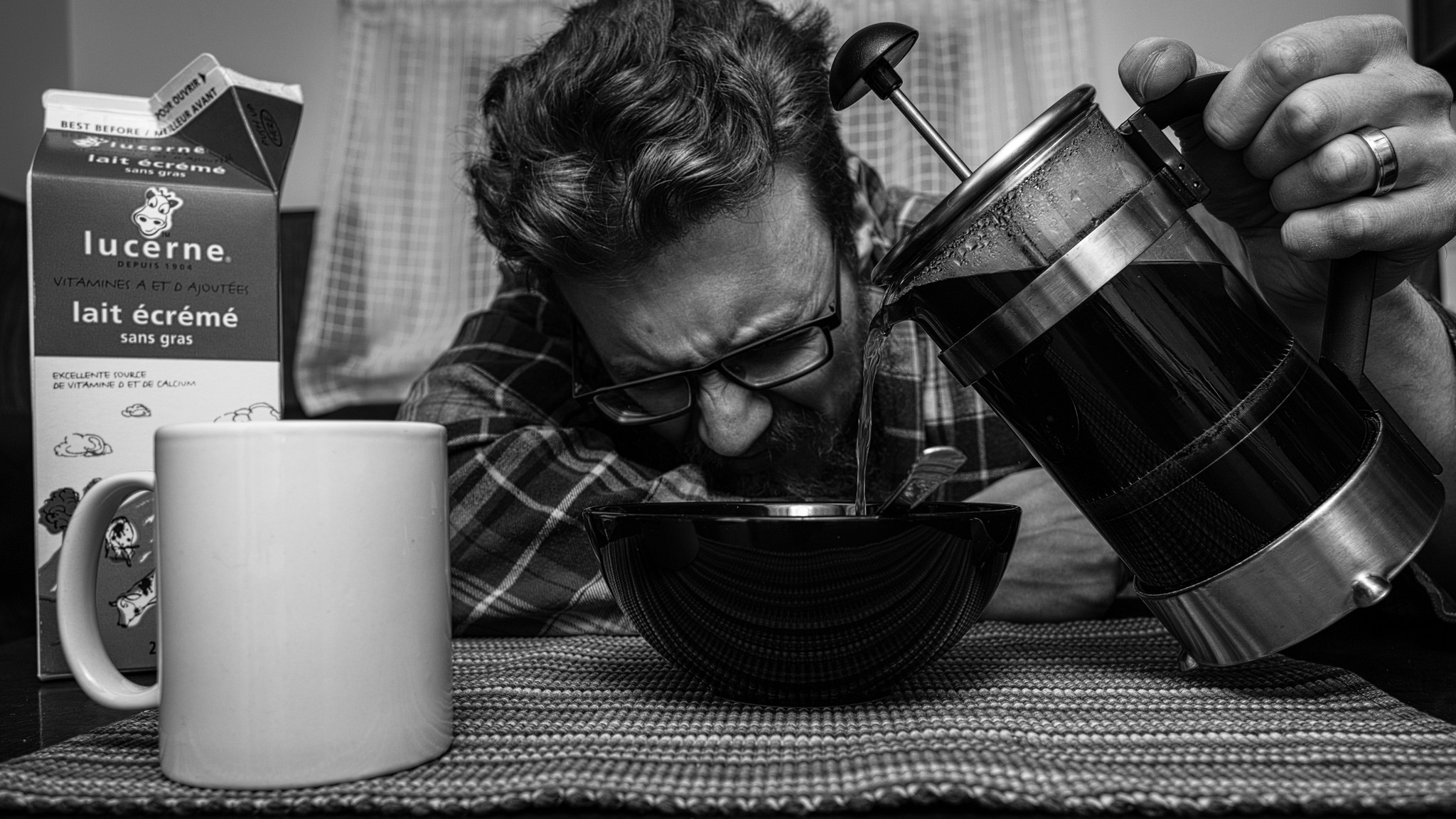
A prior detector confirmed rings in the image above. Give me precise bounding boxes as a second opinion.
[1350,126,1399,199]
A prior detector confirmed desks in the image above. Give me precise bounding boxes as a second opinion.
[0,612,1455,819]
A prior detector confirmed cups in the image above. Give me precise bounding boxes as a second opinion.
[57,413,463,787]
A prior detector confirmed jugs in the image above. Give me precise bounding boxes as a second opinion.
[829,14,1444,674]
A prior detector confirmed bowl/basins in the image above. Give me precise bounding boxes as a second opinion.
[577,492,1019,706]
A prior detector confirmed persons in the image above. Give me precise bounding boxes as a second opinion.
[389,0,1456,645]
